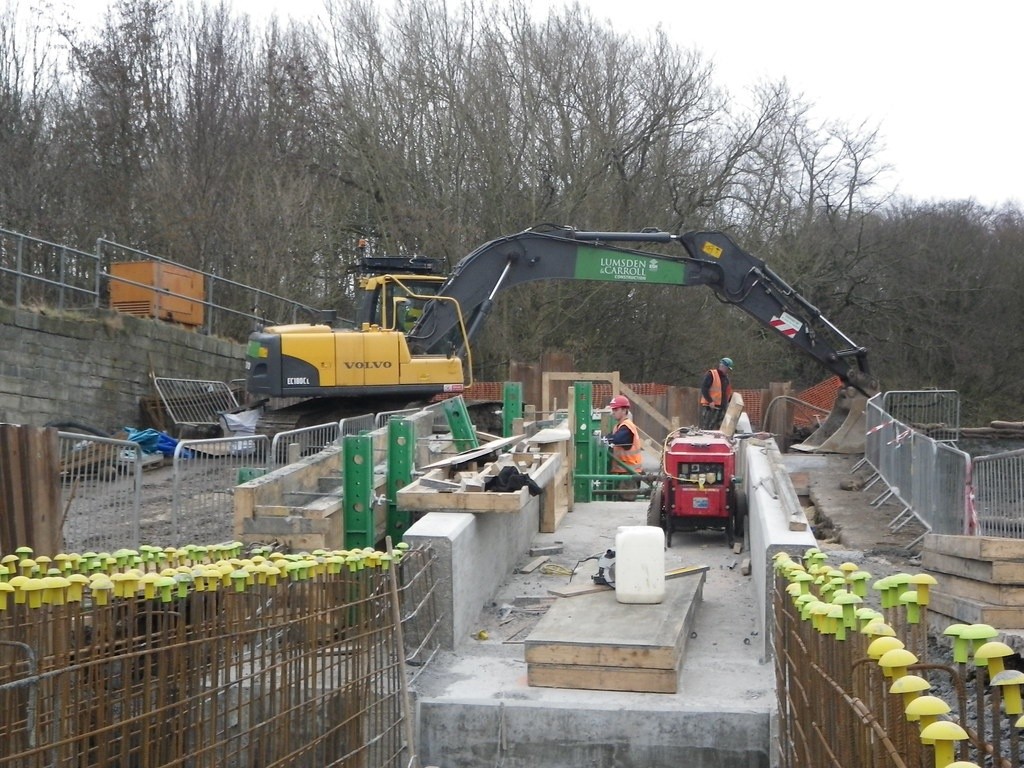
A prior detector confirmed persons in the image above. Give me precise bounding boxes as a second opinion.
[601,395,642,501]
[404,299,425,332]
[700,358,734,429]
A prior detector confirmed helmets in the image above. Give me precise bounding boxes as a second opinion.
[610,395,630,408]
[720,357,734,370]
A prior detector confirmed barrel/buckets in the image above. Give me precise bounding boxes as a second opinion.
[613,526,665,604]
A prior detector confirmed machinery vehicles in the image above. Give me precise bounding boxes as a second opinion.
[244,222,888,455]
